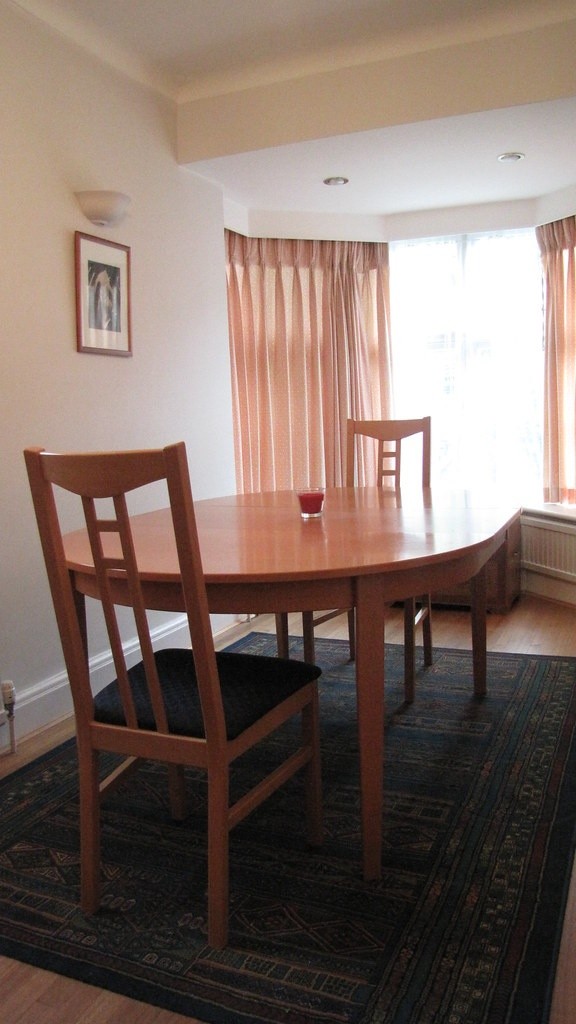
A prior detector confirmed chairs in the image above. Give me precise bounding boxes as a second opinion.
[308,415,433,703]
[22,440,323,951]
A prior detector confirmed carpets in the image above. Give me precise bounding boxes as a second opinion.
[0,631,576,1024]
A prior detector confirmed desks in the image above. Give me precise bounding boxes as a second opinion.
[53,487,524,880]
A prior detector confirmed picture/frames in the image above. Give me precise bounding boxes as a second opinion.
[68,230,133,358]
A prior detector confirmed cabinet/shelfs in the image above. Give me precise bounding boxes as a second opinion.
[392,517,521,614]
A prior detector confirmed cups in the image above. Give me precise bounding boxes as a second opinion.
[297,486,326,519]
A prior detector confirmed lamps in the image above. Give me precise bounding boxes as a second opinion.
[77,186,133,223]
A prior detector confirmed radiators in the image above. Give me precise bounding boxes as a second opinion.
[520,515,576,581]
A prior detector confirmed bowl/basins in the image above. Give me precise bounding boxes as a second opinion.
[74,189,132,227]
